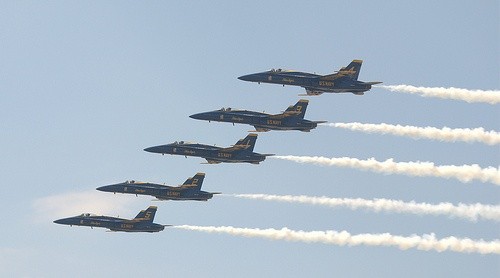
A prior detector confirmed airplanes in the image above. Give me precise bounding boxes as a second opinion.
[143,133,275,165]
[189,99,327,135]
[53,206,172,232]
[95,172,223,202]
[236,60,383,97]
